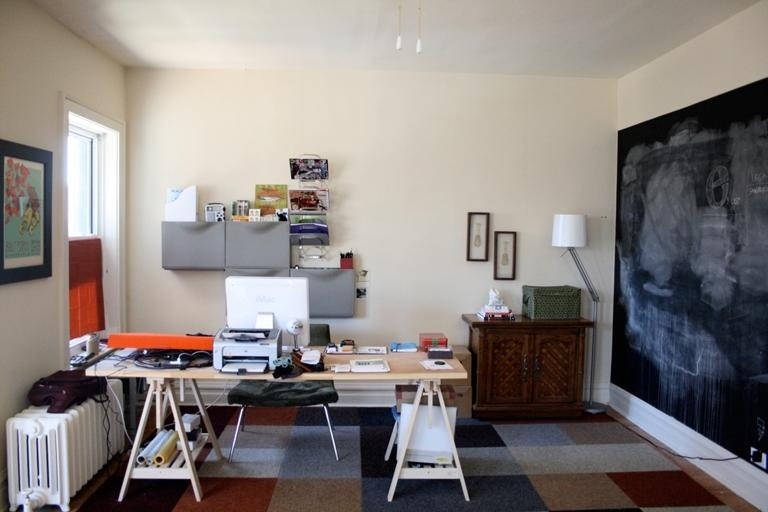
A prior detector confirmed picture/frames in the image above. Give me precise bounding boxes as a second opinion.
[0,138,53,286]
[494,230,517,282]
[468,212,489,263]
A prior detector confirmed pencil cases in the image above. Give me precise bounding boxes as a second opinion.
[428,348,453,360]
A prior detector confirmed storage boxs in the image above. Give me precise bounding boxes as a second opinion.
[523,285,581,319]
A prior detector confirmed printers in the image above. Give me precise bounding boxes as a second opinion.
[212,327,283,375]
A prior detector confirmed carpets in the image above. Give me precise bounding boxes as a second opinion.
[73,407,734,512]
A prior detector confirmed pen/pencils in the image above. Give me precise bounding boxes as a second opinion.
[340,249,353,258]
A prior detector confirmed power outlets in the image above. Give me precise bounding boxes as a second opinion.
[750,445,768,471]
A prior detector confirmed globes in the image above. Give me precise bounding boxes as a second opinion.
[286,317,304,351]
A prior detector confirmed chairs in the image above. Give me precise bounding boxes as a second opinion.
[227,324,340,463]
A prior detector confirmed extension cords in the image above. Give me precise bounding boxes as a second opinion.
[159,360,193,369]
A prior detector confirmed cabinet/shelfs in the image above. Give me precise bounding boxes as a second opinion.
[462,316,595,420]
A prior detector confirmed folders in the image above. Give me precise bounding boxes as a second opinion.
[390,342,417,352]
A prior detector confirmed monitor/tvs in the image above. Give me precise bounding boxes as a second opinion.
[225,275,311,346]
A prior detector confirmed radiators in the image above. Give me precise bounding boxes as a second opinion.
[5,376,126,512]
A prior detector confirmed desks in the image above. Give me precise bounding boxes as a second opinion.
[84,344,472,503]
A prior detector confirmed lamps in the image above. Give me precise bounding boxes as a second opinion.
[552,213,607,415]
[415,0,422,55]
[395,0,403,50]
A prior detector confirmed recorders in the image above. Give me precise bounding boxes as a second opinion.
[204,203,225,223]
